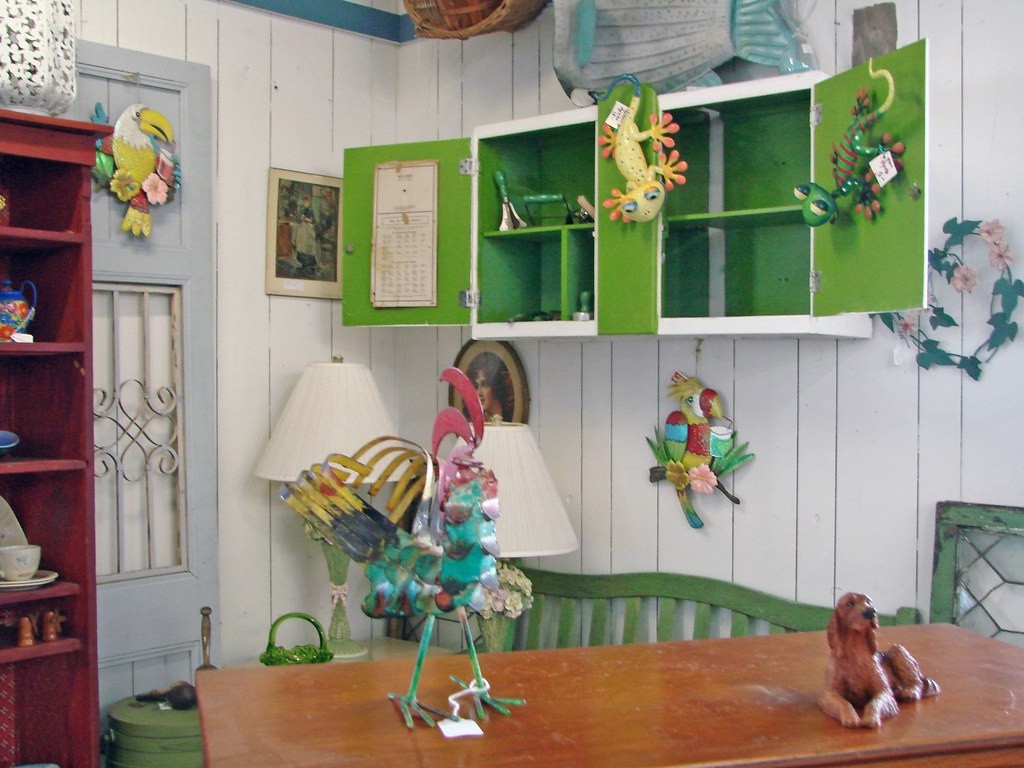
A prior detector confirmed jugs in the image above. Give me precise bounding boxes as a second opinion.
[0,279,38,343]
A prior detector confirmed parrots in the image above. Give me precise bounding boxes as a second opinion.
[648,372,756,528]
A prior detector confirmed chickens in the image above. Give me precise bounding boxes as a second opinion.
[287,365,527,728]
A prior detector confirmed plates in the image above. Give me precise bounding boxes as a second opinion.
[0,570,59,591]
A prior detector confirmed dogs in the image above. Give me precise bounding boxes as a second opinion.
[816,590,939,730]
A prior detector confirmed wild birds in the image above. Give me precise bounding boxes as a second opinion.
[90,102,183,236]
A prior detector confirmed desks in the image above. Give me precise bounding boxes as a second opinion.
[221,634,457,670]
[194,621,1024,768]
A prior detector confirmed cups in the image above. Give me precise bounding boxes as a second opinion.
[0,544,41,581]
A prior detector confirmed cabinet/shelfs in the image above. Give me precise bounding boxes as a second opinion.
[0,107,113,768]
[340,35,926,340]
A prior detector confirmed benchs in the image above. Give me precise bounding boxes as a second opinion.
[474,564,921,654]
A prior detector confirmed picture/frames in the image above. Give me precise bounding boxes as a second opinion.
[266,167,344,300]
[447,337,532,424]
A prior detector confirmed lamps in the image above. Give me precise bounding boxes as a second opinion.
[255,354,418,661]
[430,414,580,651]
